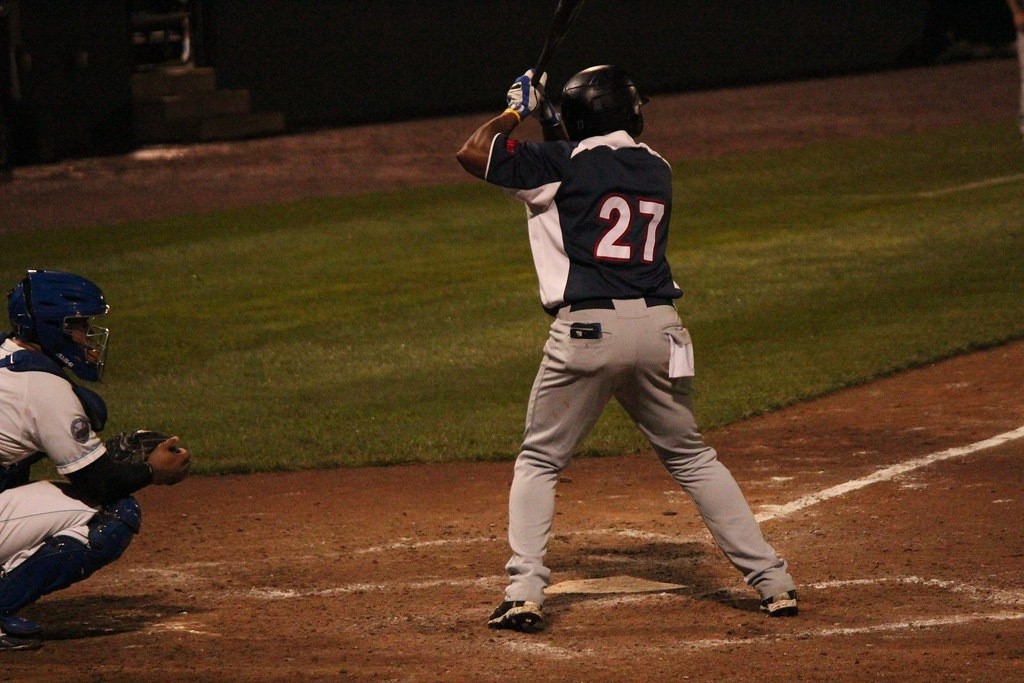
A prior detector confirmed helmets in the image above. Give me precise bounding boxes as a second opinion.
[7,270,110,382]
[560,65,650,138]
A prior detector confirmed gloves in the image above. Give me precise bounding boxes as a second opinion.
[504,68,547,123]
[530,91,562,127]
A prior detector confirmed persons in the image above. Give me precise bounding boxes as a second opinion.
[0,268,194,652]
[456,63,804,626]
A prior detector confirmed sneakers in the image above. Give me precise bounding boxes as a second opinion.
[759,589,798,613]
[0,610,44,651]
[487,599,544,634]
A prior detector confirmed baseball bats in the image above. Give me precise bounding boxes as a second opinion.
[531,0,585,85]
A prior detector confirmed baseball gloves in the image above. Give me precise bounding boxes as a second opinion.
[103,428,179,464]
